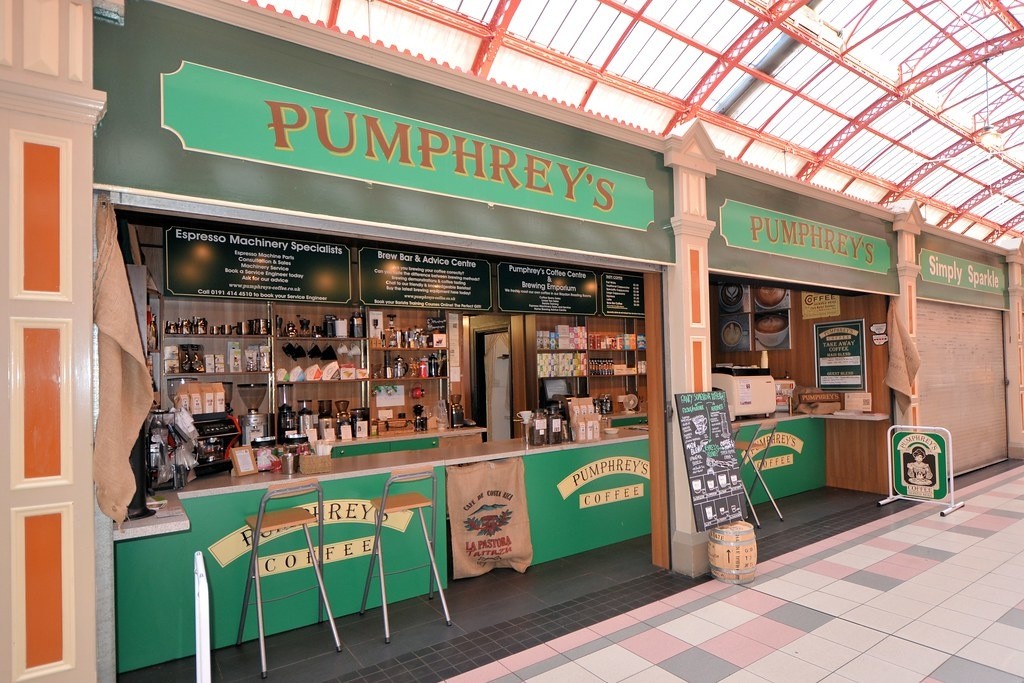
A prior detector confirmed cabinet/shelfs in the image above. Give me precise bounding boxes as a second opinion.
[525,313,648,413]
[153,294,490,448]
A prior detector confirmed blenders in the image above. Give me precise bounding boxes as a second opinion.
[167,377,269,450]
[277,384,352,446]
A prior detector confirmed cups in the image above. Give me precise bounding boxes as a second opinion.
[210,318,272,335]
[719,279,744,308]
[282,342,362,360]
[755,326,789,347]
[516,411,531,422]
[370,364,383,379]
[721,321,743,347]
[165,316,208,335]
[755,289,787,310]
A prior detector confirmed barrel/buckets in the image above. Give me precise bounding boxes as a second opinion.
[707,521,757,585]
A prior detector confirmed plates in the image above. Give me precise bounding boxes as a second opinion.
[604,429,619,434]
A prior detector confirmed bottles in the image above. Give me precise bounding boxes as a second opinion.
[386,353,439,379]
[151,449,160,483]
[146,317,160,406]
[588,358,614,376]
[761,351,769,368]
[415,411,427,432]
[529,407,570,449]
[271,443,314,475]
[573,350,587,376]
[593,394,613,414]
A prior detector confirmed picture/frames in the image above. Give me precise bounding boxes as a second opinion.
[231,445,260,477]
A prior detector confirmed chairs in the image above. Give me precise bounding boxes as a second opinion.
[734,418,785,526]
[234,479,343,676]
[361,465,452,641]
